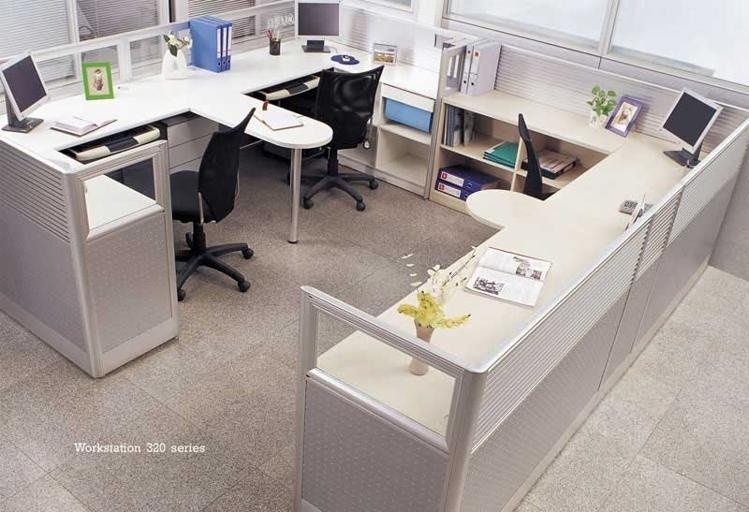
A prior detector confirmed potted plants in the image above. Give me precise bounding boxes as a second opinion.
[585,85,619,130]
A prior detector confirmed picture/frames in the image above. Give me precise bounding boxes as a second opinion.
[605,95,645,139]
[82,62,114,101]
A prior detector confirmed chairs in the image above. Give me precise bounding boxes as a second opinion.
[286,64,386,213]
[517,112,555,201]
[170,107,258,303]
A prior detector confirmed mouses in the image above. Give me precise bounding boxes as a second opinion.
[341,55,351,62]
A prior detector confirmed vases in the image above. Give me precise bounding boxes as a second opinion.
[162,50,188,80]
[406,326,435,376]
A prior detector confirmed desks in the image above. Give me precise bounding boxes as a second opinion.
[2,40,431,383]
[298,89,716,511]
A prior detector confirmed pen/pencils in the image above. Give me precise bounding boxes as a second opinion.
[264,24,284,42]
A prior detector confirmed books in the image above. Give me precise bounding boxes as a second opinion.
[464,247,551,309]
[253,108,303,130]
[49,111,117,137]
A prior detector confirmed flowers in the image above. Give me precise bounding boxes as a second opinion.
[161,30,192,59]
[395,241,480,329]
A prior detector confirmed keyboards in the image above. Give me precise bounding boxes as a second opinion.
[263,75,313,93]
[75,125,150,153]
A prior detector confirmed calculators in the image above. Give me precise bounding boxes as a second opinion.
[618,200,653,217]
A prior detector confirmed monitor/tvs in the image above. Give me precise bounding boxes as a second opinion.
[295,0,342,52]
[660,87,724,166]
[0,51,50,133]
